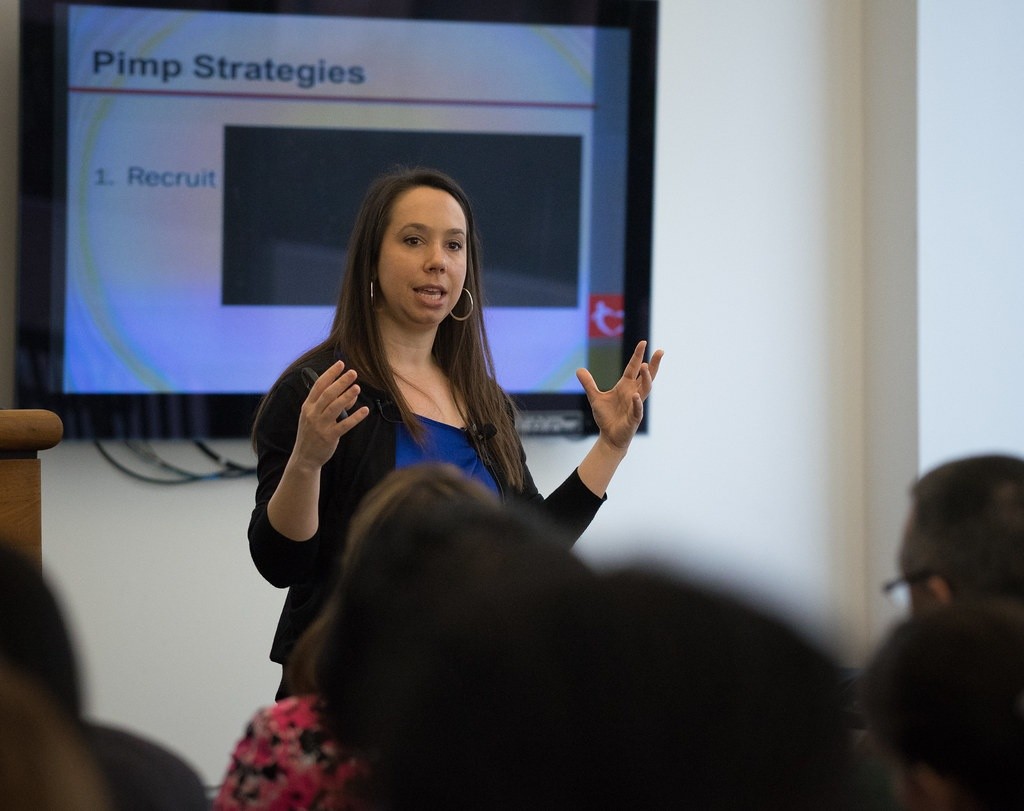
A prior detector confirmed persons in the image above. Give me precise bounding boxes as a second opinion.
[247,170,664,703]
[0,543,207,811]
[881,456,1024,618]
[213,466,899,811]
[860,594,1024,811]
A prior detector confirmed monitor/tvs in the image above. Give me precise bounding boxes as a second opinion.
[9,0,661,443]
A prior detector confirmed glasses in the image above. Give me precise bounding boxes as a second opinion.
[883,570,956,611]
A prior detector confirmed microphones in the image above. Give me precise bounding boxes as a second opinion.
[477,423,498,441]
